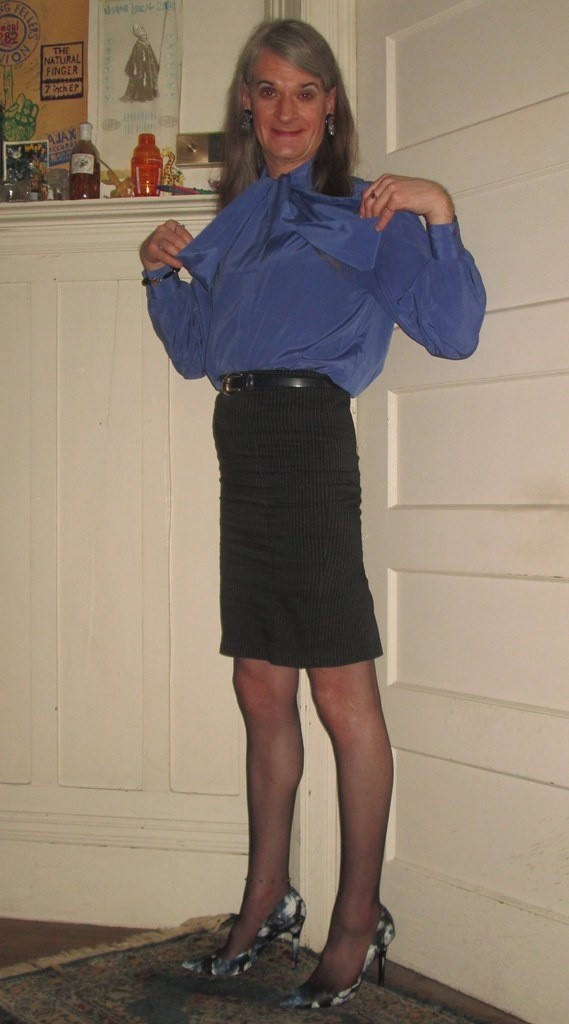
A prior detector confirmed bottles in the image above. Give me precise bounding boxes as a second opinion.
[131,134,163,197]
[25,163,41,201]
[69,122,100,200]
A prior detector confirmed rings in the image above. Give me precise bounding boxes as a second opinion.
[385,207,392,213]
[174,223,185,233]
[159,240,167,249]
[370,193,378,199]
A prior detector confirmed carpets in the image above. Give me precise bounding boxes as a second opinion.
[1,914,474,1024]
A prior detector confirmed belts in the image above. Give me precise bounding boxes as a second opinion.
[220,368,348,394]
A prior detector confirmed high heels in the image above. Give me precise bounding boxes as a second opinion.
[277,904,395,1008]
[174,888,307,983]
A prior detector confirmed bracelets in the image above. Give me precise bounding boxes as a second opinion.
[142,268,180,286]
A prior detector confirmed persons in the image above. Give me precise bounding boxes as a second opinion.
[138,20,487,1009]
